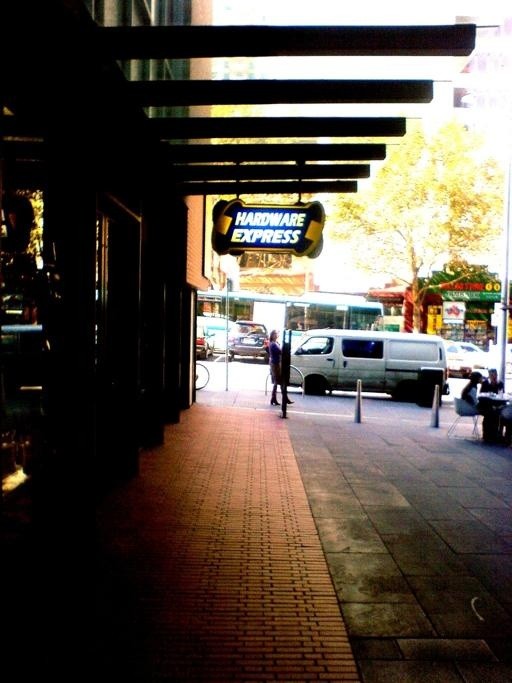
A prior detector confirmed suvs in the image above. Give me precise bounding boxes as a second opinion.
[228,321,270,362]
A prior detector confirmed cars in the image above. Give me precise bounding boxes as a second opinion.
[196,325,215,359]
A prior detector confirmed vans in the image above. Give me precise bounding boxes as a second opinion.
[289,328,449,399]
[197,316,234,352]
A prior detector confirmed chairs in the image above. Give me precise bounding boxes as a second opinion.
[322,339,332,353]
[446,397,512,448]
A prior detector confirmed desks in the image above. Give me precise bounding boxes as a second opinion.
[478,394,510,443]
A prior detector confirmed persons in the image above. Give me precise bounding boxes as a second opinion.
[296,320,302,331]
[269,330,294,405]
[462,371,483,406]
[480,369,505,444]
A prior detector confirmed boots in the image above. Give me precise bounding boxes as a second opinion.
[271,390,294,405]
